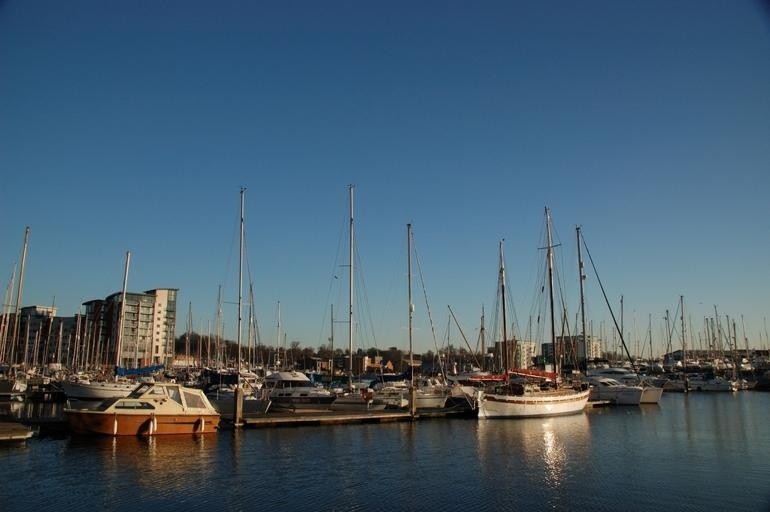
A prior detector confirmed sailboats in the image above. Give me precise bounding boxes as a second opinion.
[303,183,769,420]
[0,226,288,417]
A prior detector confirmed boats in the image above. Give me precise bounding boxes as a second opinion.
[62,382,222,437]
[262,370,337,411]
